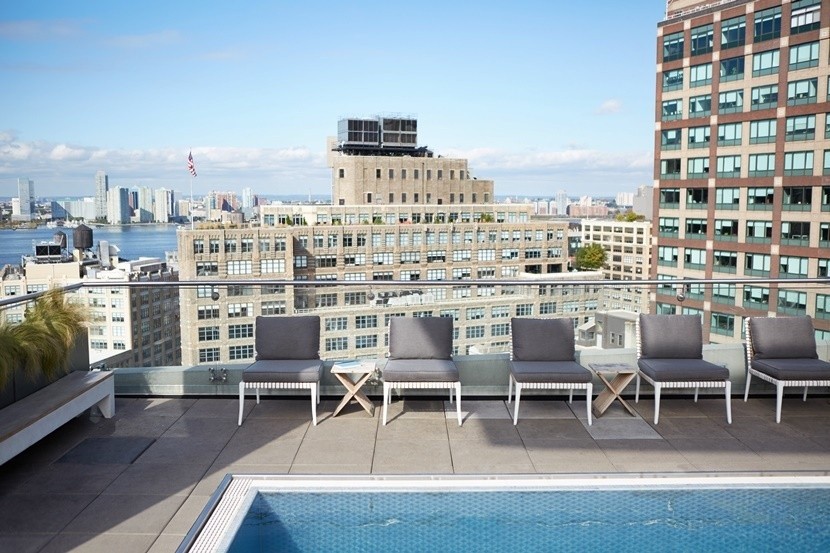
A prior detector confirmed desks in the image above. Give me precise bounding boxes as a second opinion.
[331,361,375,417]
[588,362,639,418]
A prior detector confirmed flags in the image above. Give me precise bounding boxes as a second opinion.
[188,152,197,177]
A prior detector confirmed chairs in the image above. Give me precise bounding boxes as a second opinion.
[508,316,593,427]
[237,314,324,426]
[634,312,734,425]
[743,315,830,426]
[382,316,463,426]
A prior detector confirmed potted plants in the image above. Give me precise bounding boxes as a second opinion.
[0,278,100,406]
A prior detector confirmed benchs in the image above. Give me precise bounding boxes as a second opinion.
[0,369,116,468]
